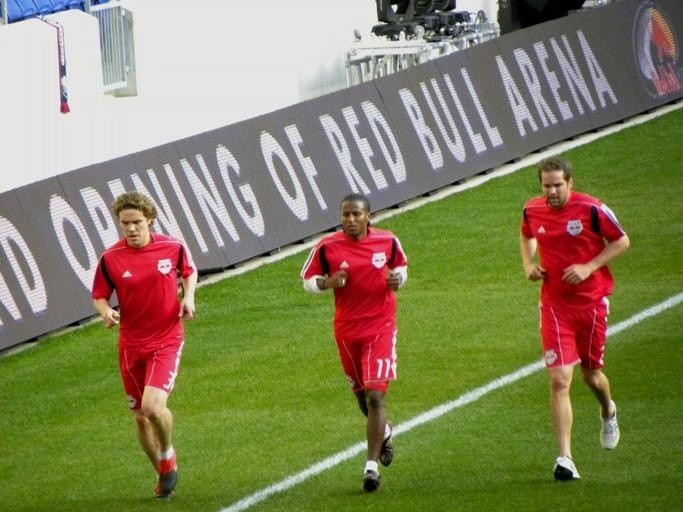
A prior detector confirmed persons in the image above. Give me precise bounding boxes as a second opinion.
[520,157,630,480]
[301,195,407,492]
[91,192,198,502]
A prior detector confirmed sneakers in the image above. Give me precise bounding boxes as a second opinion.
[377,420,393,467]
[600,400,620,450]
[362,469,380,492]
[153,446,179,501]
[552,456,580,480]
